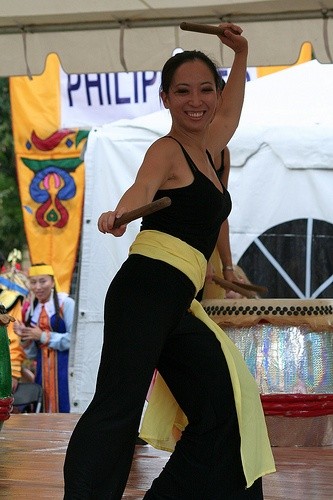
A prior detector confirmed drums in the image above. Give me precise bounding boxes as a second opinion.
[198,298,333,446]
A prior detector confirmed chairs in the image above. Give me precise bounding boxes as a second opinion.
[12,383,44,413]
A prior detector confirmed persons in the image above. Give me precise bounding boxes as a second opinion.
[138,76,277,500]
[13,263,76,413]
[225,266,260,300]
[63,23,248,500]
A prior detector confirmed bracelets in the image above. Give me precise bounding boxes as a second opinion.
[222,266,233,271]
